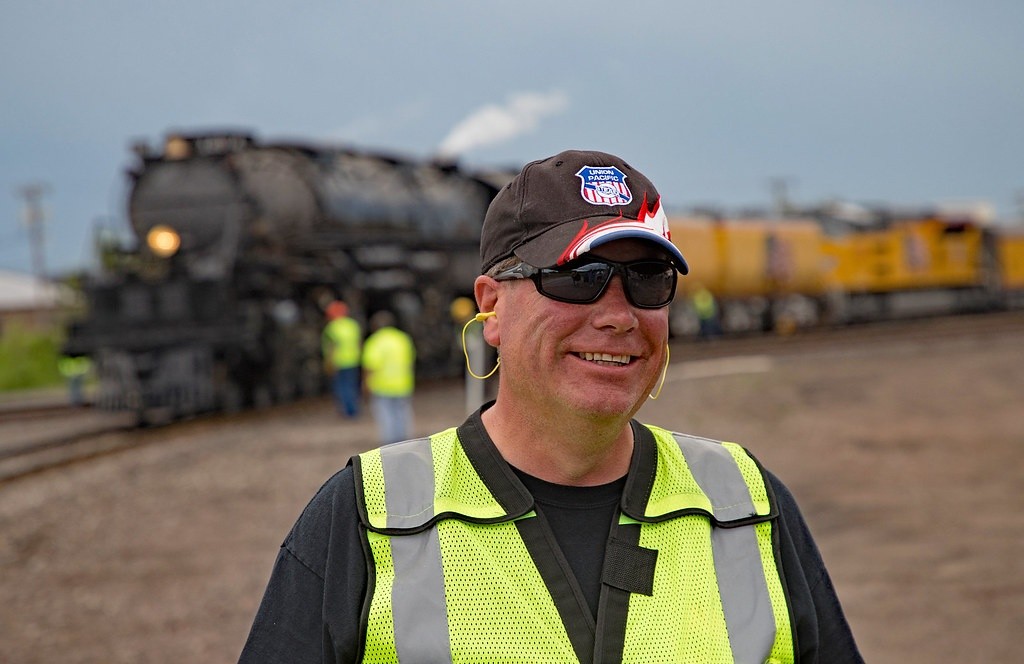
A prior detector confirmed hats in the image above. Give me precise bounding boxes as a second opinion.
[478,149,690,276]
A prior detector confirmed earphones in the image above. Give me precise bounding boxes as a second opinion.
[476,310,496,322]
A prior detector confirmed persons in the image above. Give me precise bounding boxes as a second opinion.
[322,300,362,418]
[242,150,863,664]
[360,310,415,447]
[448,297,495,406]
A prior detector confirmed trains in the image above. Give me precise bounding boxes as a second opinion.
[47,120,1024,434]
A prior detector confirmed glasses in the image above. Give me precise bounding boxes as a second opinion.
[492,258,678,310]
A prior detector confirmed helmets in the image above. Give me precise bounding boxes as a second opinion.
[325,302,348,318]
